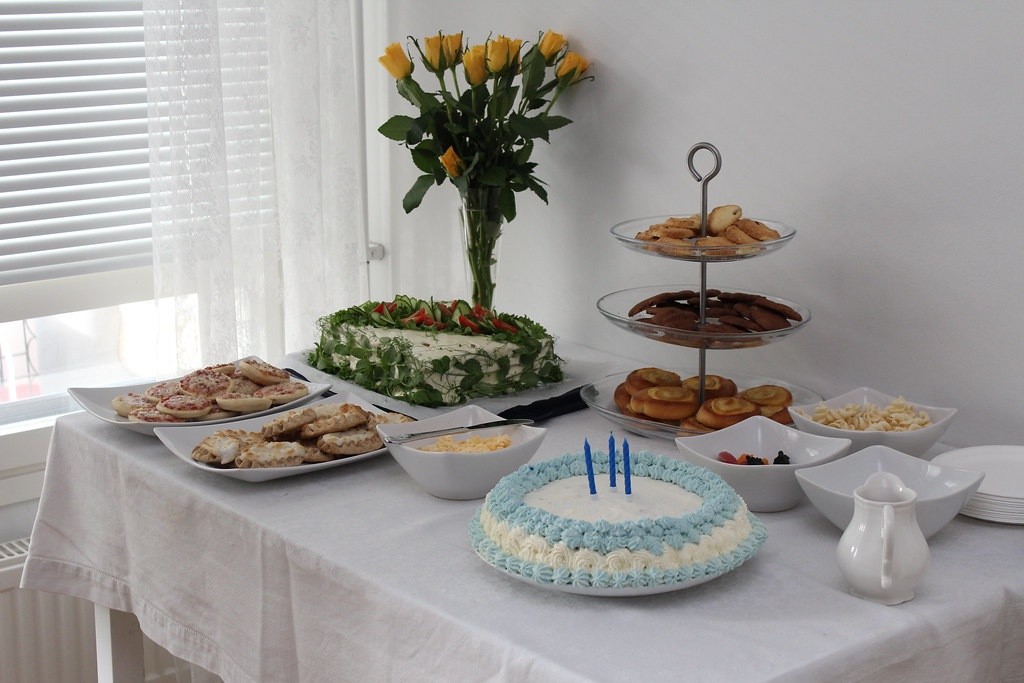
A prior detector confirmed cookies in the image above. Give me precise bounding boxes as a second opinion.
[628,288,802,342]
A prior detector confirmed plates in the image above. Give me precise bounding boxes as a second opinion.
[68,355,332,436]
[153,390,389,483]
[932,445,1024,525]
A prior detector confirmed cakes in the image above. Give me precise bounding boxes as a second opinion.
[308,295,566,409]
[469,448,767,589]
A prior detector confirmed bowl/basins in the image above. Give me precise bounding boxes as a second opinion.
[788,388,957,458]
[376,404,547,501]
[675,415,852,512]
[795,444,984,540]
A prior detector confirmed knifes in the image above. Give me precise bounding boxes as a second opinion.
[385,417,534,446]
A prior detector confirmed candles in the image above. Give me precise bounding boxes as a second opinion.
[608,431,616,488]
[622,436,631,496]
[584,438,597,495]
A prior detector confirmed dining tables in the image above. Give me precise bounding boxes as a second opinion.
[17,411,1024,682]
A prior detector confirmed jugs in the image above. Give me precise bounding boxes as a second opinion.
[838,471,931,605]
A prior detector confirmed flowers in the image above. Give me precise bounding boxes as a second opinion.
[377,30,596,310]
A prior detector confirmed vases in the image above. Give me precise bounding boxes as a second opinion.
[458,187,505,311]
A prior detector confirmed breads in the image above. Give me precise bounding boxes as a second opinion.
[614,367,798,441]
[633,204,781,257]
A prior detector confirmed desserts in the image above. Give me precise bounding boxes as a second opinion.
[112,360,416,471]
